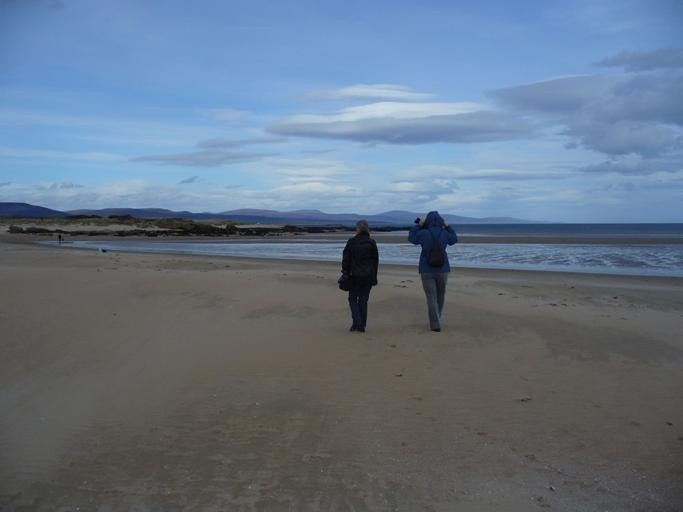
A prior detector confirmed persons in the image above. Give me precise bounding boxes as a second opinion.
[408,211,457,333]
[341,219,379,332]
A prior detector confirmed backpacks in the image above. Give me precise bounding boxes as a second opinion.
[423,228,444,267]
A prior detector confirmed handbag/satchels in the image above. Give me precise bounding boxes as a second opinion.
[339,276,354,291]
